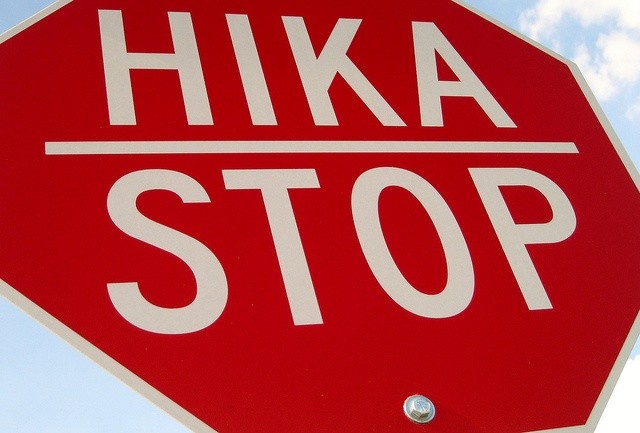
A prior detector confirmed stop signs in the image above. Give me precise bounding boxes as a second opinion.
[0,0,640,433]
